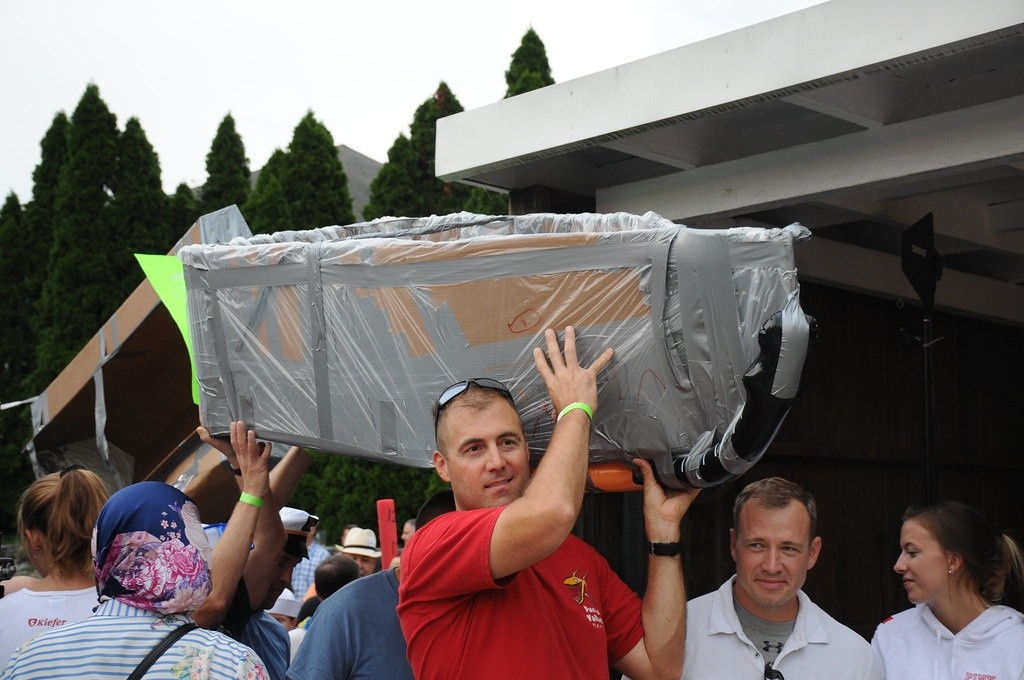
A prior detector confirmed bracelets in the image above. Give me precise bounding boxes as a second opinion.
[557,401,593,423]
[227,464,241,476]
[239,491,263,507]
[648,541,683,558]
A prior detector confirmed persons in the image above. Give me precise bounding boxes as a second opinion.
[396,326,701,680]
[621,477,884,680]
[285,490,456,680]
[871,503,1024,680]
[0,420,423,680]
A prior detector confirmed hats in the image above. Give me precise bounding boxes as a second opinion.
[278,506,319,560]
[263,588,301,618]
[334,527,382,558]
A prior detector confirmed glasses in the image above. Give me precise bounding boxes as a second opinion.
[765,662,785,680]
[435,378,515,452]
